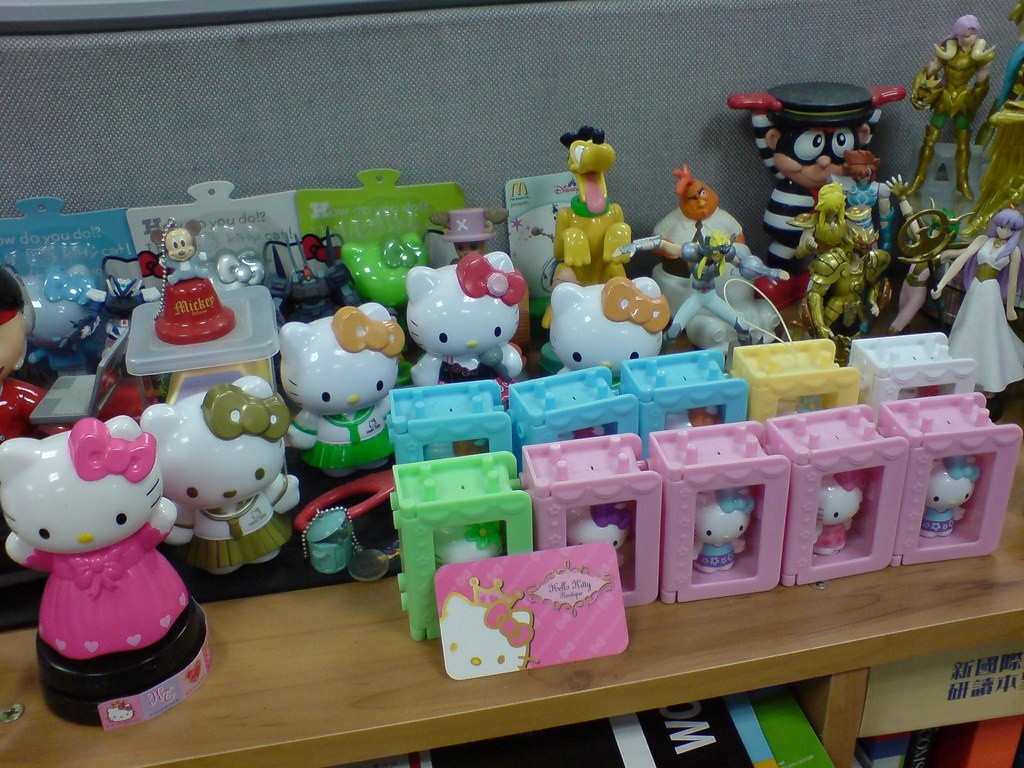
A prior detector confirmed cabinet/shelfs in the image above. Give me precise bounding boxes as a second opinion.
[0,389,1024,768]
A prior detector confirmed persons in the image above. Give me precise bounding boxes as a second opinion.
[795,149,967,367]
[906,0,1024,200]
[931,209,1024,399]
